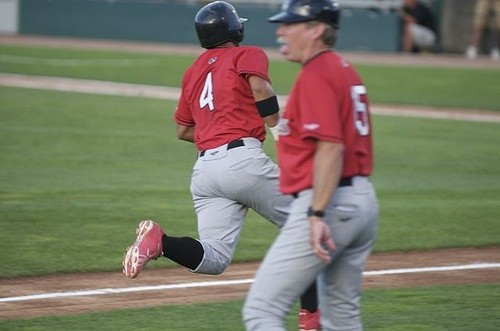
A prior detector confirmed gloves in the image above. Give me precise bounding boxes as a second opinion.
[268,117,292,141]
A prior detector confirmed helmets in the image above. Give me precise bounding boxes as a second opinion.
[267,0,342,30]
[194,1,248,48]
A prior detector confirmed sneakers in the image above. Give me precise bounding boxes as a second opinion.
[120,220,165,279]
[298,307,321,331]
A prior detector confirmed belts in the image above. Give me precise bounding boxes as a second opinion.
[200,140,245,156]
[338,176,353,187]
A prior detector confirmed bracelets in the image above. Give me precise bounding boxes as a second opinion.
[307,207,325,218]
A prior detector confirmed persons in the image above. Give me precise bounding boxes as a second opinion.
[398,0,439,53]
[242,1,377,331]
[466,0,500,61]
[122,0,321,331]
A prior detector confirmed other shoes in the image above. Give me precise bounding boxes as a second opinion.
[464,45,478,59]
[490,49,500,59]
